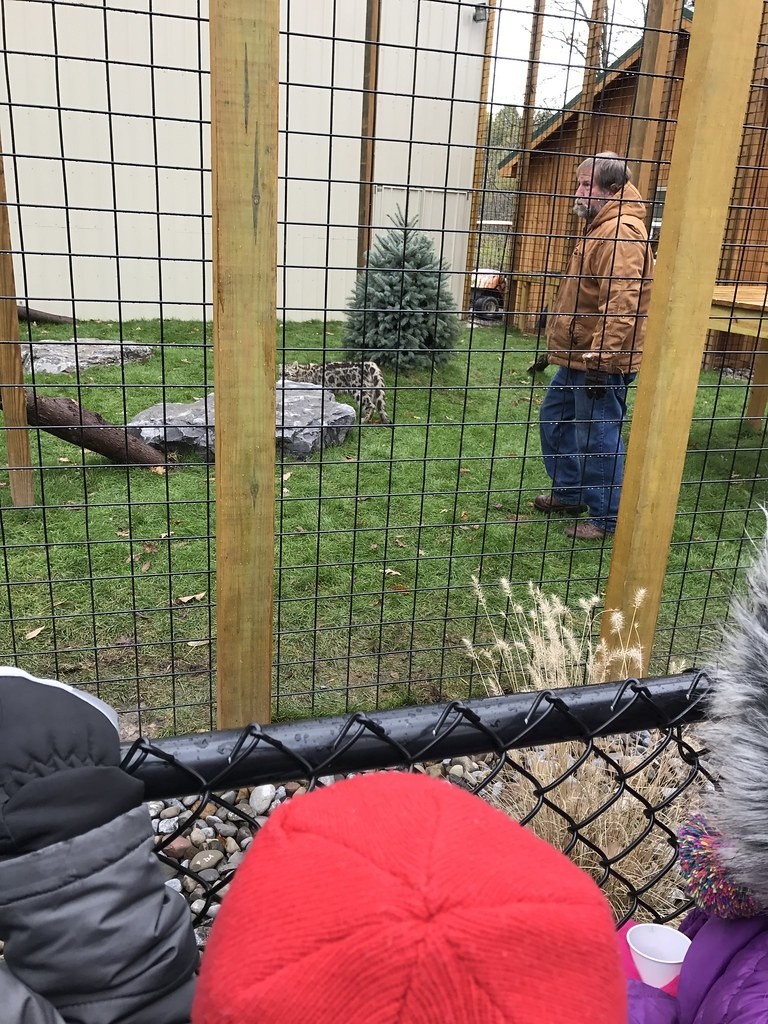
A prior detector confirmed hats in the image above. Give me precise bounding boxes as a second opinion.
[190,773,628,1024]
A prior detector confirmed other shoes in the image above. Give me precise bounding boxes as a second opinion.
[564,521,613,540]
[534,494,589,514]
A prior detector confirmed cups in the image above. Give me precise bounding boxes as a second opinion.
[626,922,692,988]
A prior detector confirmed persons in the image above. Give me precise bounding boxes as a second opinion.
[0,507,768,1024]
[533,151,655,540]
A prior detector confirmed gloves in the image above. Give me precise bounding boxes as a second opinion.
[614,917,682,1001]
[523,351,550,374]
[1,666,148,865]
[585,368,607,400]
